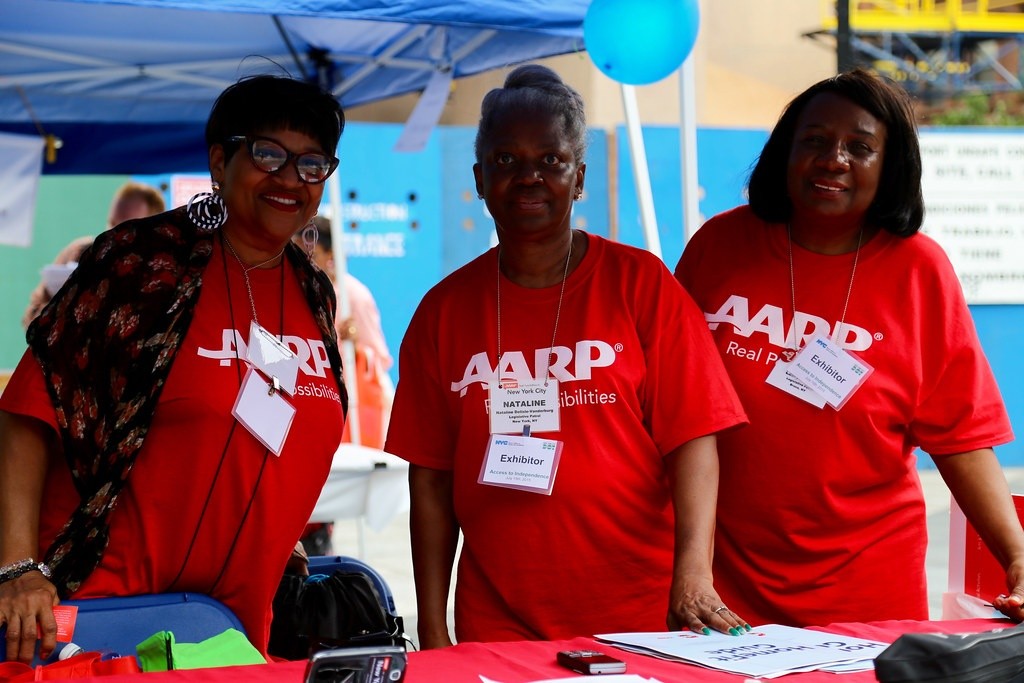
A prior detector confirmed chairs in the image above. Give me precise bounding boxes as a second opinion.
[0,592,247,668]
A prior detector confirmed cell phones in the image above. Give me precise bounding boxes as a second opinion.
[556,651,626,676]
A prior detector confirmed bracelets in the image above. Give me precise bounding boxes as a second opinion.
[0,557,52,583]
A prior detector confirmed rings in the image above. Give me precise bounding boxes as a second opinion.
[715,606,727,612]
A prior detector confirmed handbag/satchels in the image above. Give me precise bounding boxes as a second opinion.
[267,569,412,662]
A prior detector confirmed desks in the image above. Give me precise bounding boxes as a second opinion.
[307,441,409,558]
[0,618,1017,683]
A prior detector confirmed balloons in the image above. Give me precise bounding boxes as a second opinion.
[584,0,700,85]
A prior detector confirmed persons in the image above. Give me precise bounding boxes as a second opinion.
[385,61,753,650]
[673,67,1023,627]
[293,216,396,452]
[19,181,165,324]
[0,75,349,666]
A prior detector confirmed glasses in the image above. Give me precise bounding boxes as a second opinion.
[221,134,340,185]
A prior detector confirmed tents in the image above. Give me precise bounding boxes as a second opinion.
[0,0,699,263]
